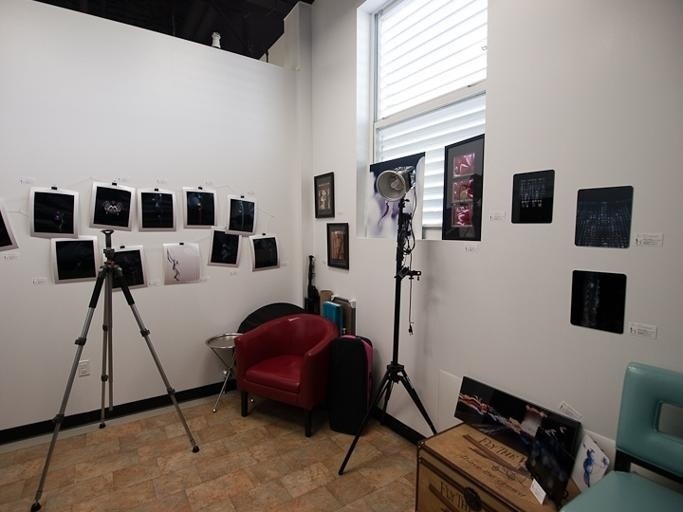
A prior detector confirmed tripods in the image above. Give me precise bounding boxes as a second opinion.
[338,199,440,476]
[30,230,198,510]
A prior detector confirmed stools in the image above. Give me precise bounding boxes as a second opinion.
[207,334,255,412]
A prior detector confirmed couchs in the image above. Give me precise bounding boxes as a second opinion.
[558,362,682,511]
[235,313,337,436]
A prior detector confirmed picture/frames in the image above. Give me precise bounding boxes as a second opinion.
[314,171,336,218]
[442,134,484,241]
[511,169,555,224]
[575,186,634,248]
[327,223,350,270]
[570,270,627,335]
[366,152,423,239]
